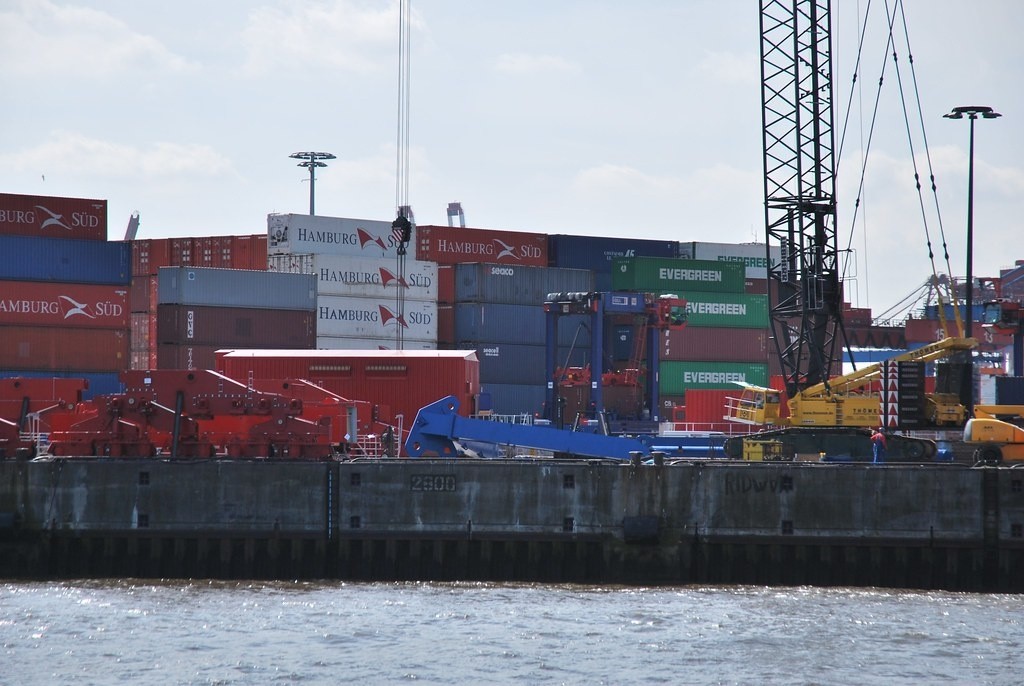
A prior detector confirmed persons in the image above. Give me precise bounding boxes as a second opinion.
[871,430,878,462]
[870,427,888,467]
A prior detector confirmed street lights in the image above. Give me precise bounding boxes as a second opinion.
[942,105,1002,418]
[288,151,337,215]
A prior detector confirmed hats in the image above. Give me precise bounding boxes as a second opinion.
[879,427,885,433]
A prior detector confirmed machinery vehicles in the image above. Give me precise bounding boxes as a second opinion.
[0,1,1024,468]
